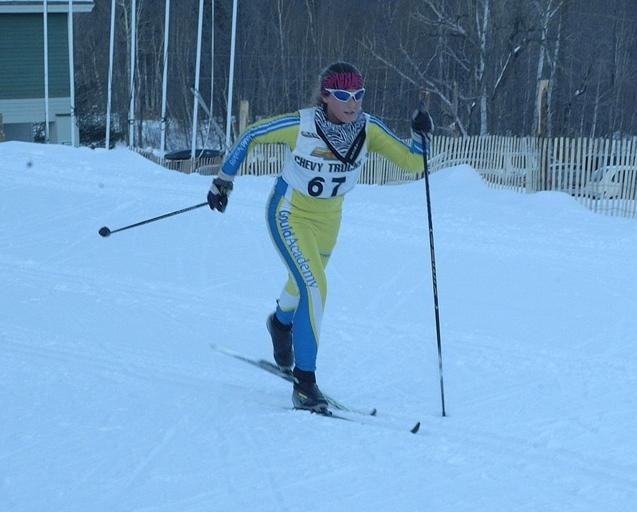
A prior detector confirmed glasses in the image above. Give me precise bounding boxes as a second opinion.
[324,87,366,104]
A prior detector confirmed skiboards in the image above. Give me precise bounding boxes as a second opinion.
[209,340,420,434]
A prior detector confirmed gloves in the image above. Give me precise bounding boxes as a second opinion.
[410,102,435,136]
[206,176,233,213]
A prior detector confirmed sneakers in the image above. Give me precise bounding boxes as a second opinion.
[291,377,329,409]
[266,311,296,369]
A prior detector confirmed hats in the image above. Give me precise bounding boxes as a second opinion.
[320,61,364,93]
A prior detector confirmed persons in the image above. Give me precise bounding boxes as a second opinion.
[205,60,438,413]
